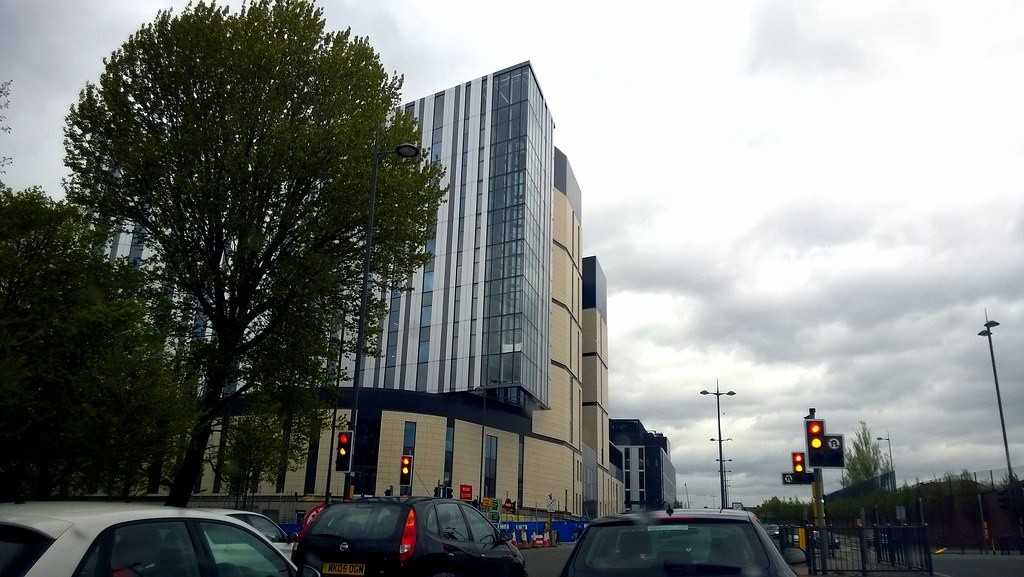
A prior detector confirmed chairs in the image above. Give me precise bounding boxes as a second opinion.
[116,525,164,577]
[655,550,692,564]
[378,514,396,536]
[165,528,201,577]
[709,538,748,565]
[612,532,653,570]
[346,521,367,537]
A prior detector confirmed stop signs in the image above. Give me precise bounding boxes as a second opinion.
[505,498,512,511]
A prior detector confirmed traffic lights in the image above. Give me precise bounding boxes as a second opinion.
[400,455,413,486]
[793,452,806,474]
[805,419,825,467]
[336,431,354,473]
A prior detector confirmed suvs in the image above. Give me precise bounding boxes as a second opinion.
[558,503,809,577]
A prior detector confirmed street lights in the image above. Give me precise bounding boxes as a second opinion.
[700,377,736,509]
[877,431,893,470]
[978,308,1013,478]
[343,130,419,497]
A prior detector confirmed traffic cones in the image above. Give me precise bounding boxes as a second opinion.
[542,528,552,548]
[511,527,518,546]
[521,526,529,547]
[531,528,538,548]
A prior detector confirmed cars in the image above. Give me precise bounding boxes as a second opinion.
[762,522,840,548]
[0,501,323,577]
[186,506,297,561]
[291,497,529,577]
